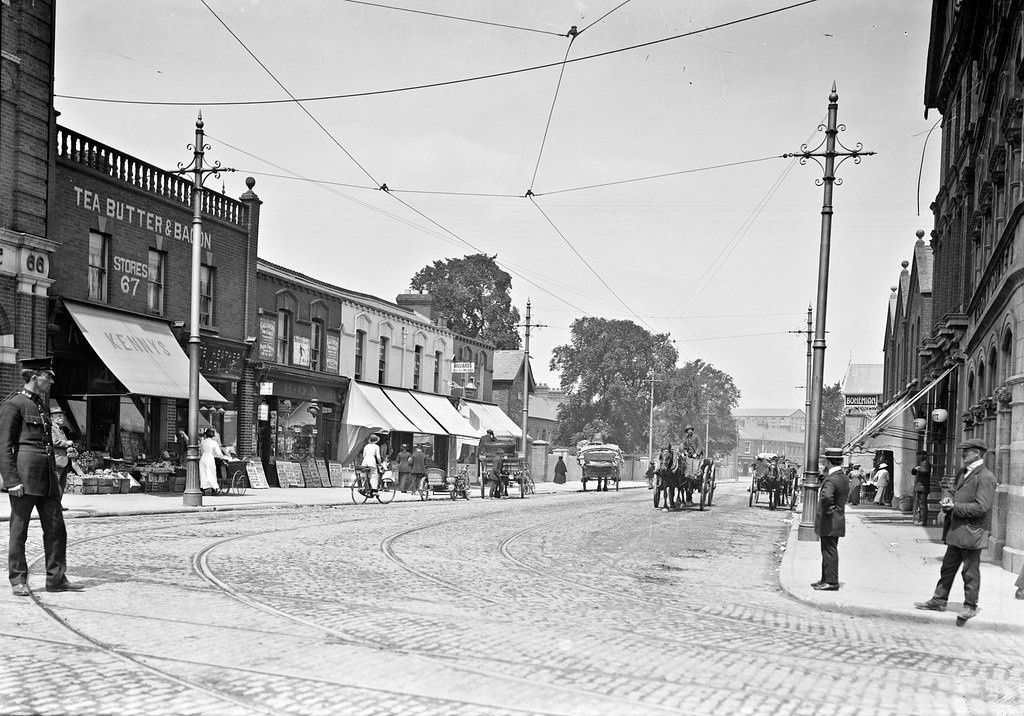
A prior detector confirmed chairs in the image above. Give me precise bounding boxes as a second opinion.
[425,468,446,486]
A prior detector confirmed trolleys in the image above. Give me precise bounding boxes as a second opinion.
[205,456,251,496]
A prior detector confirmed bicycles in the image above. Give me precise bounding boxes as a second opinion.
[520,462,536,499]
[350,456,396,505]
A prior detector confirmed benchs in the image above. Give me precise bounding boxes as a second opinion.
[583,453,616,467]
[482,444,519,457]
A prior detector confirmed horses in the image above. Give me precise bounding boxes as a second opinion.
[658,443,692,512]
[501,480,509,497]
[597,477,608,491]
[765,459,785,511]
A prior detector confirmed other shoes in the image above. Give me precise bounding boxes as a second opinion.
[500,495,508,499]
[61,506,68,511]
[372,492,380,496]
[957,605,977,620]
[490,497,497,499]
[212,490,220,495]
[914,600,944,611]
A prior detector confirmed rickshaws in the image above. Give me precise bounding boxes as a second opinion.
[417,462,471,501]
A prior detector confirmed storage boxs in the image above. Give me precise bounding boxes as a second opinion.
[68,477,130,494]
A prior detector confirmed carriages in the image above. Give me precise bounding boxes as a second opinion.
[477,435,526,499]
[575,438,625,492]
[747,451,799,511]
[652,443,717,512]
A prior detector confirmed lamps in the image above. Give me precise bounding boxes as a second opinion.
[913,403,934,430]
[931,390,957,422]
[199,405,226,415]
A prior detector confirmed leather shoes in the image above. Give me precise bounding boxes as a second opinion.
[46,580,84,592]
[813,583,839,591]
[12,583,30,596]
[811,581,824,586]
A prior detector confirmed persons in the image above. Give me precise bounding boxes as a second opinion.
[408,446,428,496]
[50,406,73,511]
[0,357,84,596]
[914,438,997,620]
[199,430,229,495]
[845,464,867,505]
[378,439,395,492]
[1015,565,1024,600]
[645,461,655,489]
[396,444,412,493]
[458,430,504,500]
[811,448,850,591]
[361,434,381,497]
[684,424,704,459]
[911,451,931,526]
[553,456,567,484]
[873,463,890,505]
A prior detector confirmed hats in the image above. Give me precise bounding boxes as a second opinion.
[878,463,888,468]
[401,444,408,449]
[49,406,65,415]
[416,443,423,449]
[368,434,380,444]
[19,356,56,376]
[957,438,988,450]
[685,425,694,432]
[853,465,859,469]
[820,447,847,458]
[917,450,927,454]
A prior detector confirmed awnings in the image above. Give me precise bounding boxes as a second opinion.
[842,362,959,454]
[457,397,532,461]
[61,298,231,403]
[336,378,481,468]
